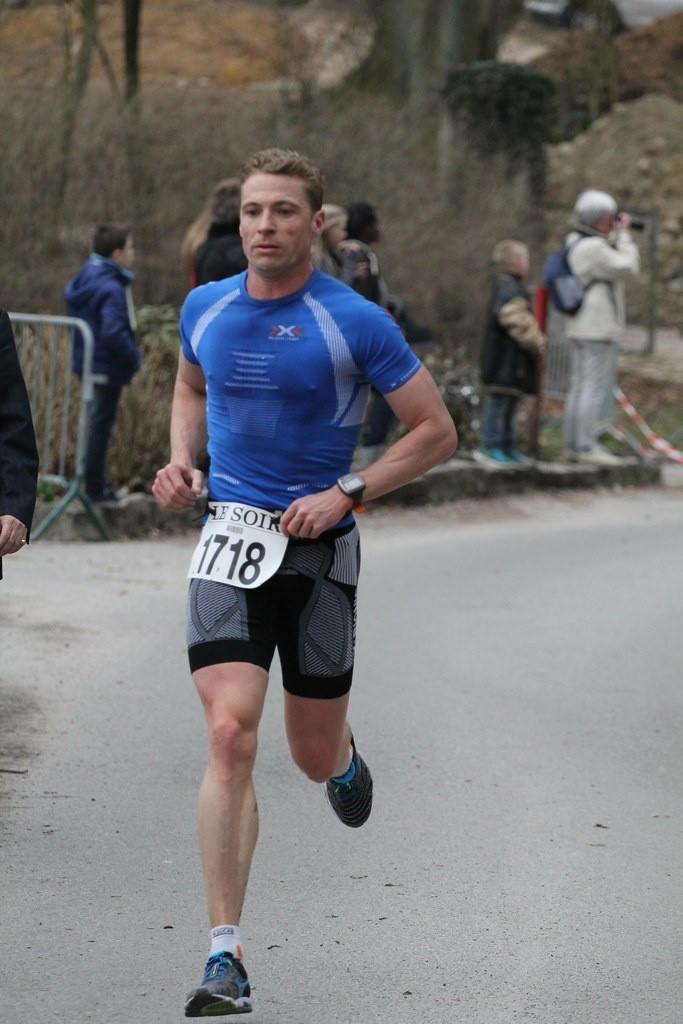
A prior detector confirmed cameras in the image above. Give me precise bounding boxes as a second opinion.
[614,215,643,230]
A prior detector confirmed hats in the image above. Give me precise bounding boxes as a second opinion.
[321,203,348,228]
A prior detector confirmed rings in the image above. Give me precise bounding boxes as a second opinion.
[21,540,27,544]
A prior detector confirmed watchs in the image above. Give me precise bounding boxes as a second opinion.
[336,471,367,514]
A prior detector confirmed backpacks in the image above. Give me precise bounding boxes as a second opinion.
[543,230,599,314]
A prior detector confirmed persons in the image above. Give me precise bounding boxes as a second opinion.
[473,239,548,469]
[151,147,459,1017]
[187,177,406,454]
[65,223,142,504]
[561,190,644,466]
[0,306,40,556]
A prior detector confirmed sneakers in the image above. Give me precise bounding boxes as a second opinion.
[184,949,252,1017]
[323,732,373,828]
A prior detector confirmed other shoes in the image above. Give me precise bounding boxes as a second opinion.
[561,446,618,466]
[473,446,514,470]
[85,482,129,508]
[360,443,383,469]
[507,449,534,471]
